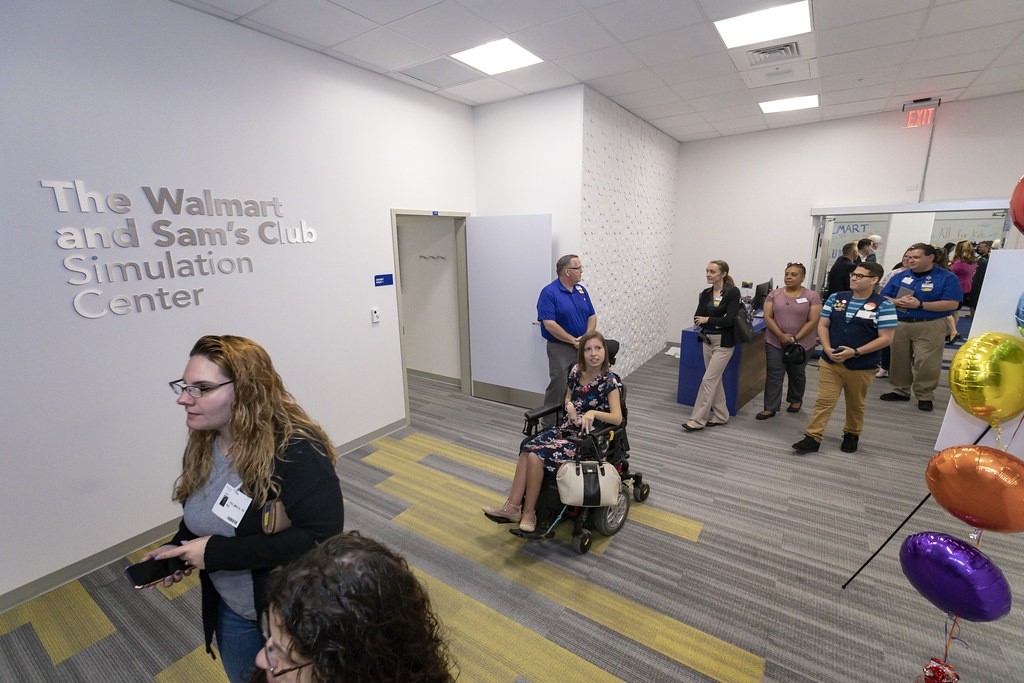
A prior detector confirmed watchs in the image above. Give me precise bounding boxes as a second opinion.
[918,300,923,311]
[852,347,861,359]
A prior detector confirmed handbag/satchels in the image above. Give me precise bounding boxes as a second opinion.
[733,303,754,344]
[783,336,807,364]
[556,435,622,508]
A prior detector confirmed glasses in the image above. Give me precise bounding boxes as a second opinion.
[261,607,314,677]
[168,378,234,399]
[566,266,584,270]
[787,262,803,266]
[849,272,876,280]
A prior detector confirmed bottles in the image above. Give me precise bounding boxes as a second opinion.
[740,280,755,315]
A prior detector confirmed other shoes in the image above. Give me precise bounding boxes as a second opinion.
[706,421,727,427]
[519,510,537,532]
[875,368,888,378]
[786,401,803,413]
[756,410,776,420]
[918,400,933,411]
[480,504,522,523]
[880,392,910,401]
[682,423,703,431]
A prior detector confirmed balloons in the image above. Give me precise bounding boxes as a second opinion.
[948,331,1024,429]
[899,531,1012,623]
[1009,175,1024,234]
[1015,292,1024,337]
[924,445,1024,532]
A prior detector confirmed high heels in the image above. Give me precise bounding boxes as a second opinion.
[945,333,961,345]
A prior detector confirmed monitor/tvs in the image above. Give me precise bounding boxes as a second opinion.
[753,278,774,310]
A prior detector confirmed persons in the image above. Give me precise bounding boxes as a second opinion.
[879,244,963,411]
[536,254,597,431]
[481,330,624,533]
[254,530,460,683]
[136,334,345,683]
[827,235,993,379]
[755,262,822,421]
[791,262,899,454]
[681,260,741,431]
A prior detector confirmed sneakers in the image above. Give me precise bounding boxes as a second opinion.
[841,433,859,453]
[792,434,820,453]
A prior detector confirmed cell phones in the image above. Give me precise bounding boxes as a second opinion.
[124,554,196,590]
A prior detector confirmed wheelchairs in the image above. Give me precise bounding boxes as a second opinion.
[484,339,650,554]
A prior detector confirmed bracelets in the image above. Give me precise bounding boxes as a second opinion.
[564,402,575,412]
[793,336,799,343]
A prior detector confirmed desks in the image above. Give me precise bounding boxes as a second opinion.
[677,310,767,417]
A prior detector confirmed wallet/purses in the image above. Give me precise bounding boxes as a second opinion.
[262,499,292,534]
